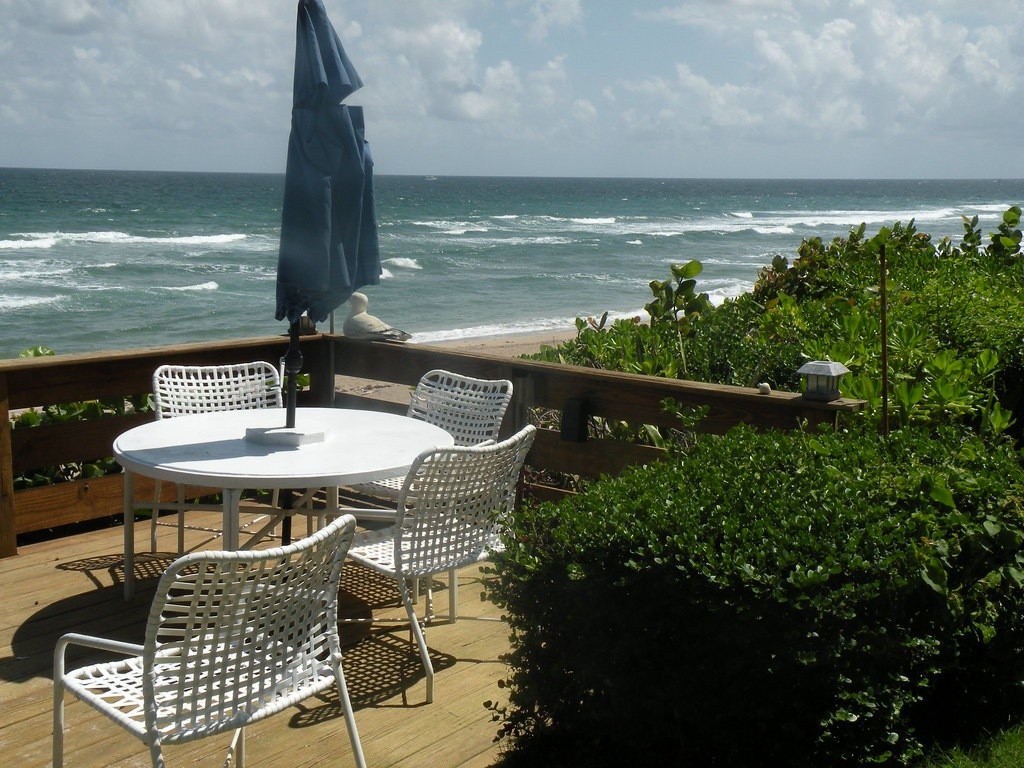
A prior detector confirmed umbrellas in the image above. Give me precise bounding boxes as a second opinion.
[275,0,382,582]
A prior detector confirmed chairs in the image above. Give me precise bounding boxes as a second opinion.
[343,369,514,604]
[53,514,368,768]
[330,423,537,704]
[151,362,302,569]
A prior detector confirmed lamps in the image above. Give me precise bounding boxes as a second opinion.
[287,311,319,334]
[795,360,850,402]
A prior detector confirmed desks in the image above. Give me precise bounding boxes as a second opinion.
[112,407,458,626]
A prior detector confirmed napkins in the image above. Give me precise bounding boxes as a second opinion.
[244,428,304,448]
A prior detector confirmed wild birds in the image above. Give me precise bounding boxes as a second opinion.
[343,292,412,340]
